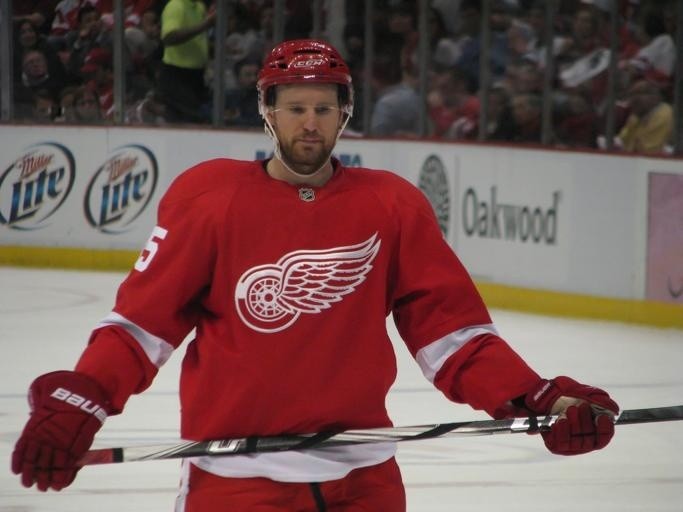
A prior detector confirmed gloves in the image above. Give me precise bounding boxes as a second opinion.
[526,376,619,455]
[12,371,111,491]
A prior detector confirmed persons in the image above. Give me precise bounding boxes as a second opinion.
[10,0,683,154]
[9,40,617,512]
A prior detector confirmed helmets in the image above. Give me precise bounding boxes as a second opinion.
[257,40,355,117]
